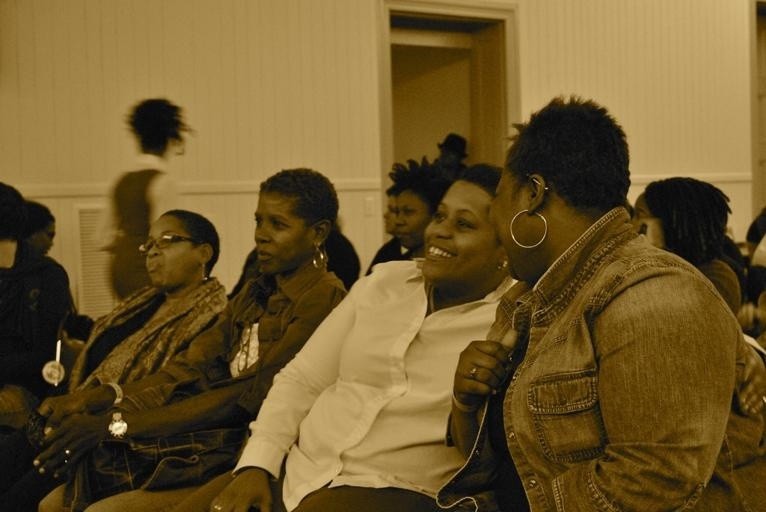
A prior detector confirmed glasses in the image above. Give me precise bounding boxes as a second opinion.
[138,233,200,254]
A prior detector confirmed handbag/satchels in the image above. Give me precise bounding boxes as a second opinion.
[69,427,249,511]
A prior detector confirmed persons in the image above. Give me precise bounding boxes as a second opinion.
[0,95,766,512]
